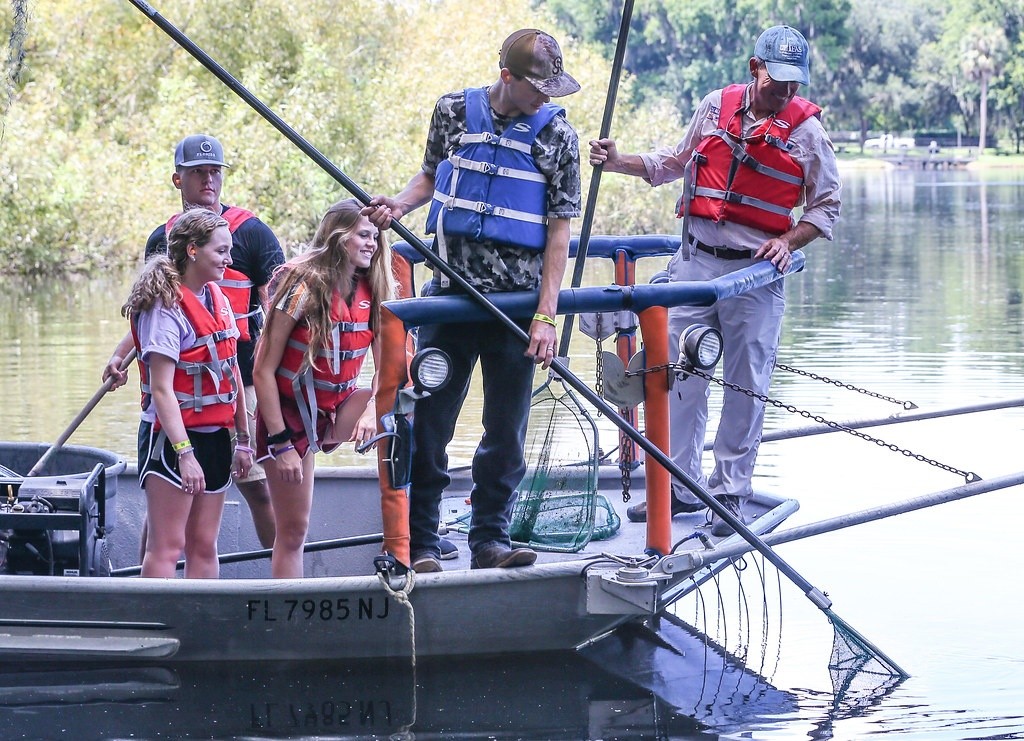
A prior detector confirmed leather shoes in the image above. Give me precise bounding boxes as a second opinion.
[470,545,538,569]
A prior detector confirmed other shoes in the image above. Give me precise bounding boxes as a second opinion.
[436,539,459,560]
[412,554,443,573]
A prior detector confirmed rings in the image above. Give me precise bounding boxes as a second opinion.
[187,484,193,487]
[548,348,554,352]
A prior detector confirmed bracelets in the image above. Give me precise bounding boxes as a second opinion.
[177,447,194,460]
[367,396,375,406]
[235,433,251,442]
[172,439,191,451]
[234,445,254,454]
[533,313,557,327]
[274,445,294,456]
[265,429,291,446]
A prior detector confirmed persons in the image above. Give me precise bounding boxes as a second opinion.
[589,25,842,536]
[102,134,286,566]
[252,199,403,579]
[121,198,254,579]
[361,28,582,572]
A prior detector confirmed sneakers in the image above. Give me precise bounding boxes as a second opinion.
[712,494,747,536]
[627,483,708,522]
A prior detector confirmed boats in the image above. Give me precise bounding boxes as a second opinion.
[9,234,1020,740]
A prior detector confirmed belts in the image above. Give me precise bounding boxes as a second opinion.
[688,233,752,261]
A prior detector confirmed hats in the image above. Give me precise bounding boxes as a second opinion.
[174,135,230,170]
[500,29,581,97]
[755,25,811,86]
